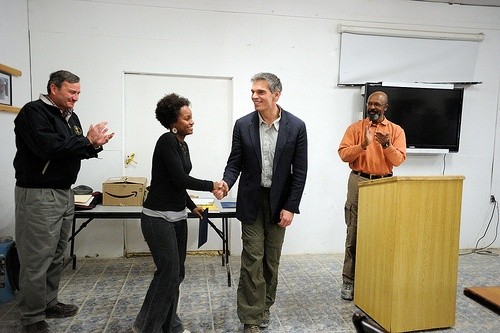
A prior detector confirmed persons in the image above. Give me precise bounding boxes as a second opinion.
[338,91,406,300]
[13,70,115,333]
[132,92,227,333]
[212,72,308,333]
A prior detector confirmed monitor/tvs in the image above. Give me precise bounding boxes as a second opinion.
[362,82,464,153]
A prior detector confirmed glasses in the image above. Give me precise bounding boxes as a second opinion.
[367,103,387,107]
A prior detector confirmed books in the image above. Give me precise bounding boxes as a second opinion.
[191,198,214,206]
[74,195,92,203]
[74,196,94,206]
[220,202,237,208]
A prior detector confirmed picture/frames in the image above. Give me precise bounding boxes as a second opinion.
[0,70,12,106]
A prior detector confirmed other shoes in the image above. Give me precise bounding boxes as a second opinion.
[341,281,354,299]
[244,324,261,333]
[45,302,79,318]
[22,320,50,333]
[259,308,271,327]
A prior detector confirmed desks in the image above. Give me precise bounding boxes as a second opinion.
[59,199,238,288]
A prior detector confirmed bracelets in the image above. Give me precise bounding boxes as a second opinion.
[382,142,389,148]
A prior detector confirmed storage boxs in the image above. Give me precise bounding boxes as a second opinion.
[102,176,147,206]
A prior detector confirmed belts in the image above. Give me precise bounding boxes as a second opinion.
[352,171,393,180]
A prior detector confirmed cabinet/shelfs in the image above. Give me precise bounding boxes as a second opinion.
[0,63,23,113]
